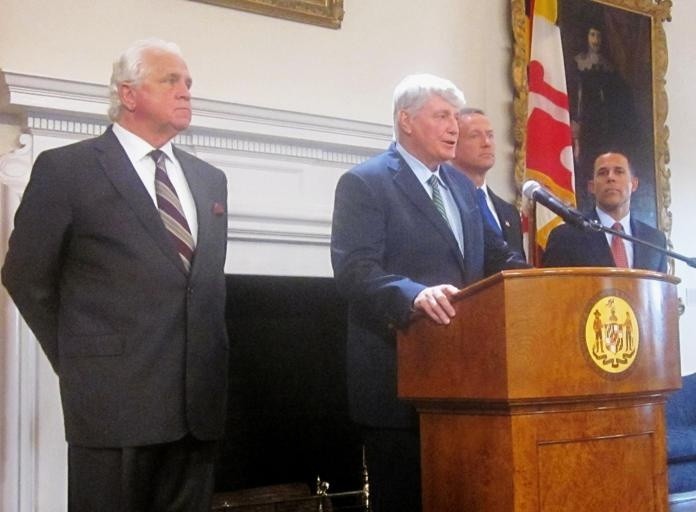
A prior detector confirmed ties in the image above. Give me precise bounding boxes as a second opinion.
[428,175,449,228]
[148,151,196,272]
[611,223,629,268]
[478,189,501,238]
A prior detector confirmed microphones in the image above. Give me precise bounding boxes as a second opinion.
[521,180,583,227]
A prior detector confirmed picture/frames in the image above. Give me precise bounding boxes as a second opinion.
[506,0,684,312]
[187,0,345,30]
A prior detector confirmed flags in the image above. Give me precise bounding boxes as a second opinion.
[526,0,579,266]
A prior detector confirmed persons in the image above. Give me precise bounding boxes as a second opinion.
[539,151,670,273]
[330,71,532,512]
[564,17,609,163]
[448,106,526,279]
[1,39,230,510]
